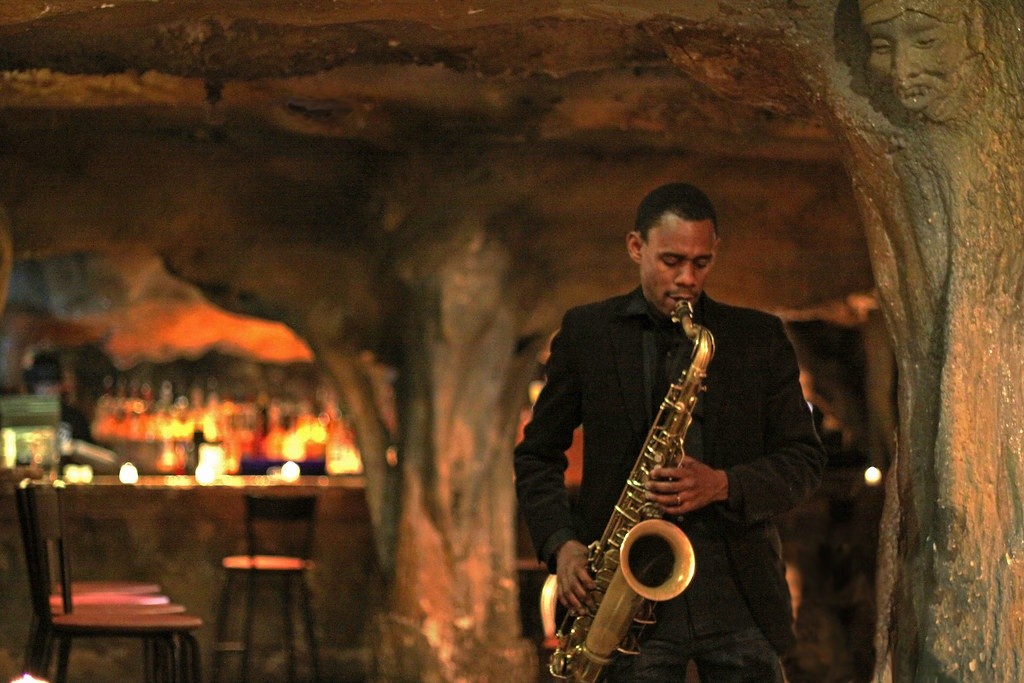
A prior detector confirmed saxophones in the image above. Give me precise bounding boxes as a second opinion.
[545,300,715,682]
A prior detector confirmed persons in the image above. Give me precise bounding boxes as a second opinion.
[862,0,980,120]
[23,355,93,476]
[512,185,832,683]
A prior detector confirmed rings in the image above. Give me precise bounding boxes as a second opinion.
[676,494,680,506]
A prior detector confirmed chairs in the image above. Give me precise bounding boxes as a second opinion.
[12,480,204,683]
[216,493,328,683]
